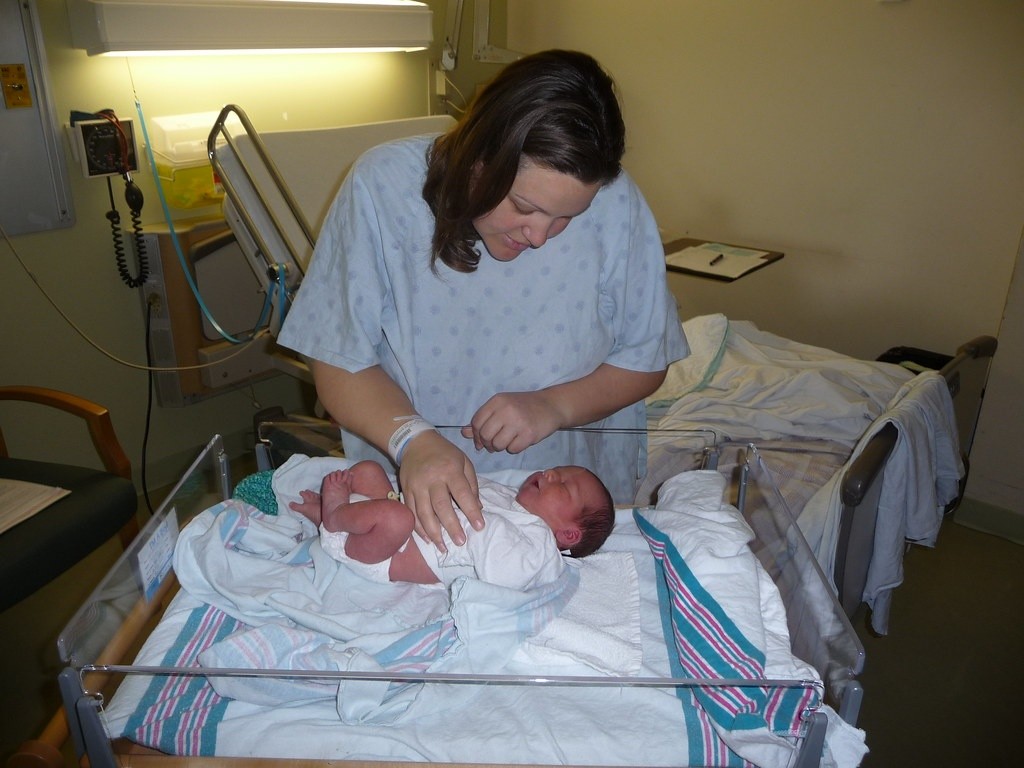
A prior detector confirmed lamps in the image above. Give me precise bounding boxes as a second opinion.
[63,0,435,58]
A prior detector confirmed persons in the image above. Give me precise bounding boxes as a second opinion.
[288,459,615,592]
[276,50,693,555]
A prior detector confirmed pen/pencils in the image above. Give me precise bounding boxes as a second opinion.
[708,253,725,265]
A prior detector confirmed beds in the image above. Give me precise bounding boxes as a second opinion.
[205,99,1001,626]
[55,422,863,767]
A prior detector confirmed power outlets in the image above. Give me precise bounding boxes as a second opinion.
[146,282,165,320]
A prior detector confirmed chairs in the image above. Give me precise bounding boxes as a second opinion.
[1,386,142,612]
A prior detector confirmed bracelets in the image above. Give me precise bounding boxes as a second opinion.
[388,415,441,468]
